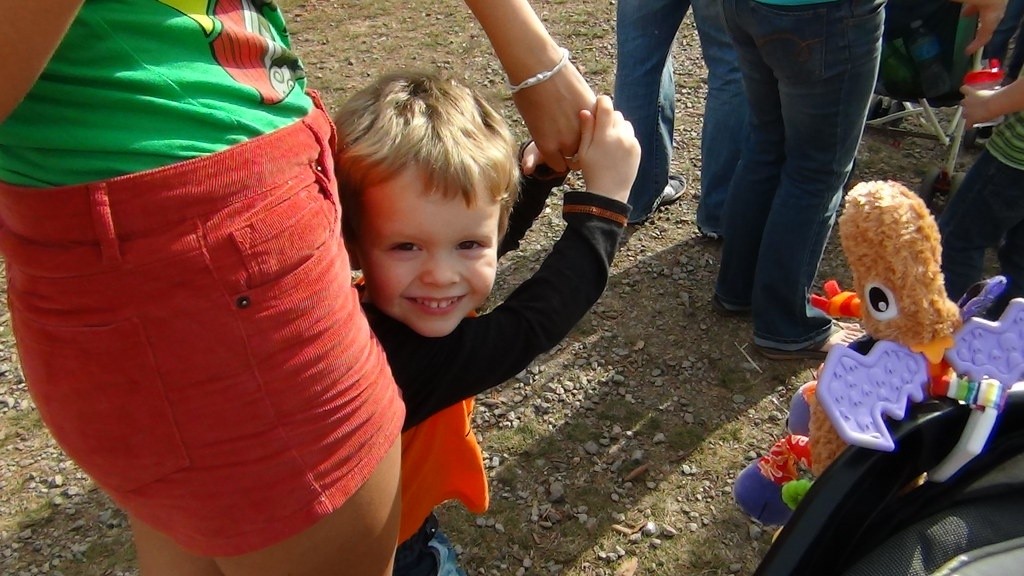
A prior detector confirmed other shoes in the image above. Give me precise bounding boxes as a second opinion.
[628,174,686,223]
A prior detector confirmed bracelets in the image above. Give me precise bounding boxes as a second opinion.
[510,44,572,94]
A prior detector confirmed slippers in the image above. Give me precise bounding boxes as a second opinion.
[713,290,867,360]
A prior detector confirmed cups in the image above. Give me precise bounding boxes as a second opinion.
[963,59,1004,128]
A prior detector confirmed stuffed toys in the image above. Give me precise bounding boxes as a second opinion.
[733,179,1024,528]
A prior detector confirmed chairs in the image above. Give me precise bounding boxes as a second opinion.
[866,0,985,178]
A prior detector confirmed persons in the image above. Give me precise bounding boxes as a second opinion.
[1,0,596,576]
[712,0,1007,361]
[333,67,642,575]
[615,0,754,246]
[982,0,1023,86]
[937,60,1024,323]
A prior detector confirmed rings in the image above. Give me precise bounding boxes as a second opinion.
[563,152,577,160]
